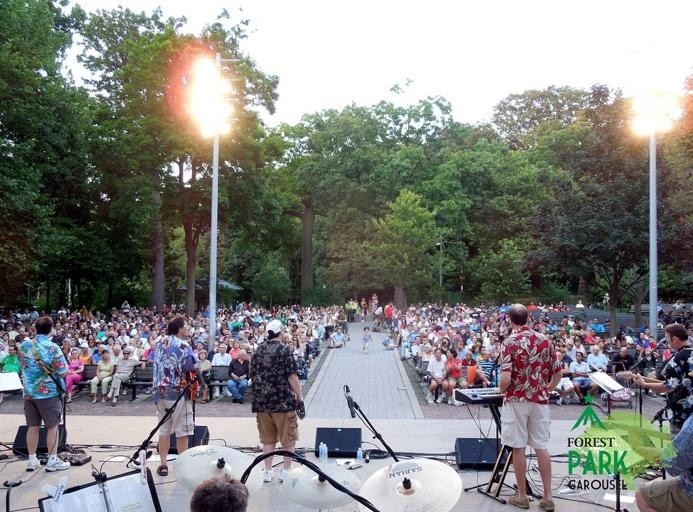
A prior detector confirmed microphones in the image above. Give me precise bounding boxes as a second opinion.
[343,385,356,418]
[193,364,206,391]
[656,338,665,347]
[666,378,691,407]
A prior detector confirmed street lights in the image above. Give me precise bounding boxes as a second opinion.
[180,56,236,347]
[625,68,686,334]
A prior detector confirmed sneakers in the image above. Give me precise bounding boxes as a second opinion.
[509,495,529,509]
[263,470,273,482]
[232,397,245,403]
[92,396,117,406]
[278,470,291,482]
[45,458,71,472]
[26,457,40,471]
[539,499,554,511]
[557,397,586,406]
[427,395,452,405]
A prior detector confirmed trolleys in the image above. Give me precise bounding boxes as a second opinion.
[603,361,633,409]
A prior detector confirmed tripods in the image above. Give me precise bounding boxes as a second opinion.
[463,366,517,493]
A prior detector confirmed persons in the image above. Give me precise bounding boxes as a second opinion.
[495,303,563,511]
[249,319,304,482]
[151,316,201,476]
[190,474,251,511]
[1,294,361,407]
[358,293,692,406]
[633,323,693,440]
[635,384,693,512]
[16,316,71,473]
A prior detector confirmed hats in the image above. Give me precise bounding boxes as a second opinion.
[266,320,284,334]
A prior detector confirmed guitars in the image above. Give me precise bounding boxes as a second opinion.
[56,374,67,399]
[615,371,666,383]
[188,371,198,401]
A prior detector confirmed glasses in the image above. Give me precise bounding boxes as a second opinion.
[124,353,129,354]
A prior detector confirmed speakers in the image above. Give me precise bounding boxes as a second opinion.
[455,438,508,470]
[12,424,67,454]
[157,426,210,454]
[315,428,362,458]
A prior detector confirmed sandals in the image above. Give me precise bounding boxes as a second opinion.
[157,466,168,476]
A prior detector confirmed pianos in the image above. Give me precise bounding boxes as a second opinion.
[455,387,559,404]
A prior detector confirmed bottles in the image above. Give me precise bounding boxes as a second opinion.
[322,443,328,462]
[357,448,362,464]
[319,442,324,461]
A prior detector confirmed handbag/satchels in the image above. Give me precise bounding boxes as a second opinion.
[452,388,466,406]
